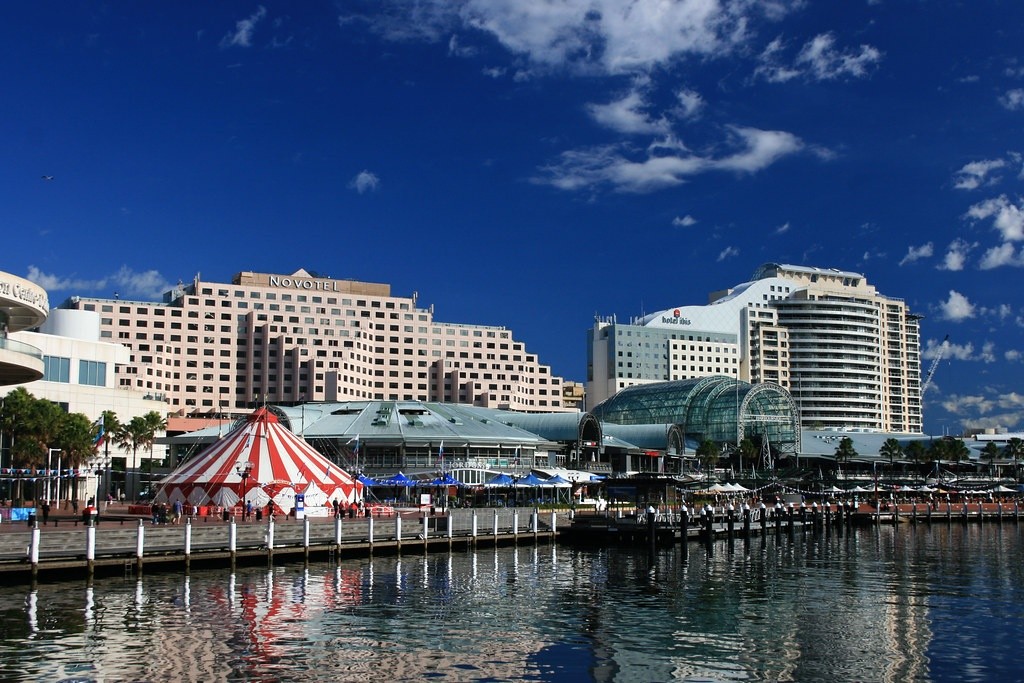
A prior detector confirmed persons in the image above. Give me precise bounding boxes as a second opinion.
[120,492,126,505]
[933,497,939,508]
[215,504,223,520]
[158,502,167,525]
[192,504,197,520]
[267,500,277,522]
[70,497,78,514]
[171,497,183,525]
[801,493,806,504]
[150,502,159,524]
[333,497,365,519]
[41,500,50,525]
[430,499,436,516]
[106,494,112,502]
[244,500,252,522]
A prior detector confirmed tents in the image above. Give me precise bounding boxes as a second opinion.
[152,405,363,518]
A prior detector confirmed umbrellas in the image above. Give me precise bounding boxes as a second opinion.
[733,482,751,492]
[482,472,513,488]
[515,472,550,488]
[822,485,844,493]
[421,472,463,505]
[352,473,381,501]
[722,482,737,492]
[892,485,916,491]
[916,486,935,492]
[702,483,727,492]
[546,474,574,489]
[937,484,1017,494]
[380,470,418,503]
[866,486,887,492]
[847,485,869,492]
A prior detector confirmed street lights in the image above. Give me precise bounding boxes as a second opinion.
[235,461,255,522]
[435,470,449,515]
[87,456,112,525]
[510,471,522,507]
[567,473,580,516]
[345,464,364,504]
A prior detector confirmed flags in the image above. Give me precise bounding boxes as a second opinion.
[89,415,104,450]
[344,434,359,460]
[434,439,443,467]
